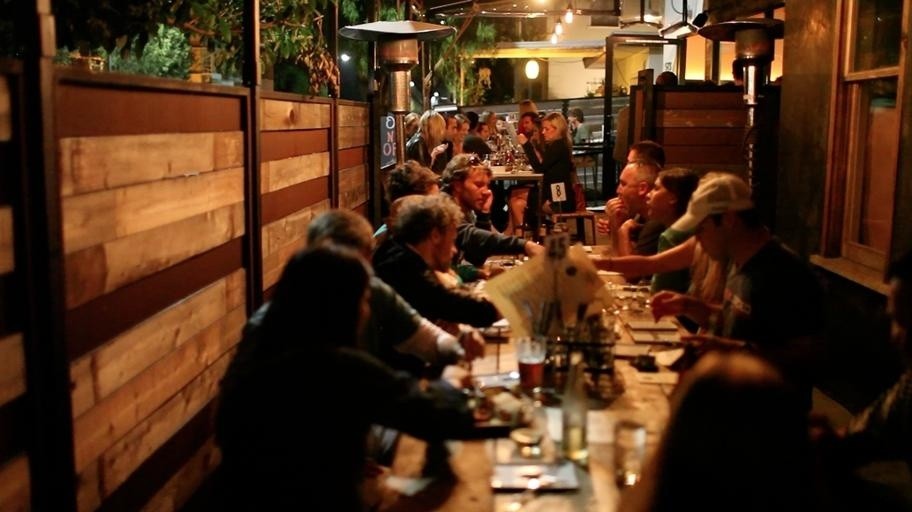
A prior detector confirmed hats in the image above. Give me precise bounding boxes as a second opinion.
[671,170,755,234]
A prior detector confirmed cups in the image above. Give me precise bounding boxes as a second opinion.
[480,138,527,173]
[613,419,647,489]
[508,399,549,450]
[490,254,515,278]
[516,334,549,389]
[601,280,654,314]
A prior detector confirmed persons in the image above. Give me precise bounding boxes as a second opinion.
[211,72,911,512]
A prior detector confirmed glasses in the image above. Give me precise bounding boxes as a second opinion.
[465,154,484,168]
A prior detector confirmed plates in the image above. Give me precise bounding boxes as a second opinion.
[490,462,581,494]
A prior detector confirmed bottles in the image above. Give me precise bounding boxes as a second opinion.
[563,355,589,468]
[512,224,548,245]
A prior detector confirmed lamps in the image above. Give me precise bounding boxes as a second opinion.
[564,0,573,24]
[554,15,563,35]
